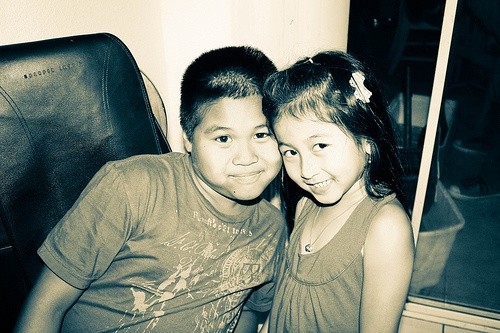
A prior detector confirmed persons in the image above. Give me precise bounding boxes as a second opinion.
[260,50,415,333]
[15,46,290,333]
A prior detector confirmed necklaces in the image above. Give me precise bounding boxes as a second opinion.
[304,194,369,253]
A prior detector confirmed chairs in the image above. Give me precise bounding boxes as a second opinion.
[0,32,258,333]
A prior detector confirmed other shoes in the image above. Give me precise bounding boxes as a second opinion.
[448,178,500,201]
